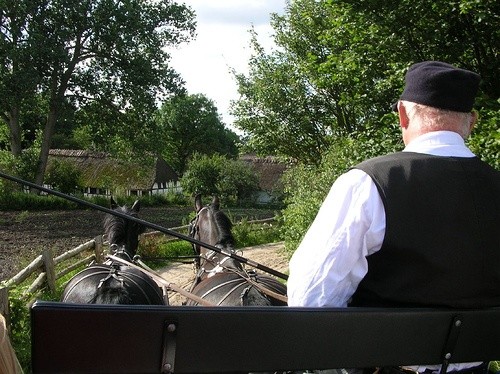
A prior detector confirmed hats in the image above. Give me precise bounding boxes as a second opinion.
[393,61,482,115]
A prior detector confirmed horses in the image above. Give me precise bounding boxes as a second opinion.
[62,196,165,306]
[181,194,288,306]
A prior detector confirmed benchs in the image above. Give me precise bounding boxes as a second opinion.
[30,301,500,374]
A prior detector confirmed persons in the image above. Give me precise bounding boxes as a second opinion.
[287,62,500,374]
